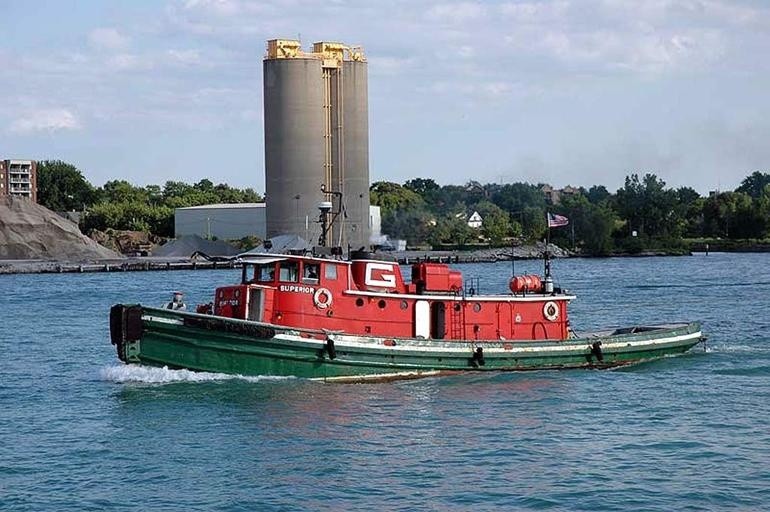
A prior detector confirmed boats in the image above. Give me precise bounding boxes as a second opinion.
[106,183,709,381]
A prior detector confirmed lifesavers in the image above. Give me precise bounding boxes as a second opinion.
[543,301,559,321]
[315,287,332,310]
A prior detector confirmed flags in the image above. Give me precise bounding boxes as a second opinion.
[546,212,570,228]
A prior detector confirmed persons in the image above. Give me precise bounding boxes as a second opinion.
[268,270,274,281]
[308,267,318,279]
